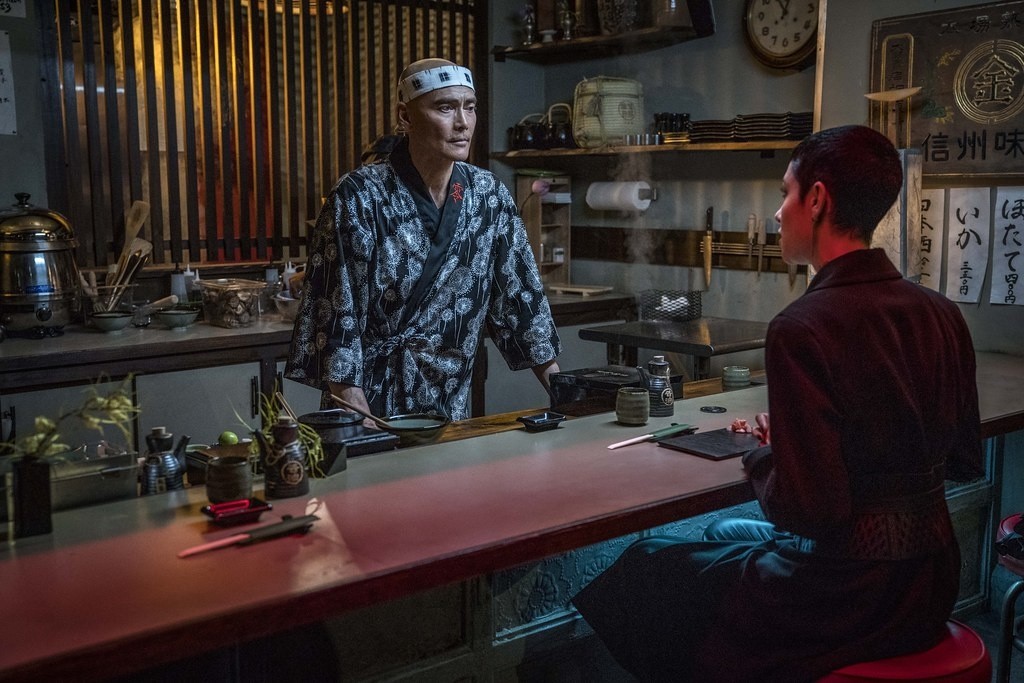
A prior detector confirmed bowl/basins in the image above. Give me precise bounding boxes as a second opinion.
[89,311,134,333]
[375,414,451,450]
[155,306,200,331]
[271,292,300,321]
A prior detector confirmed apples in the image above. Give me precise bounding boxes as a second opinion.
[219,432,239,445]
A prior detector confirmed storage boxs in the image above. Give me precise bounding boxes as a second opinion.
[549,364,650,416]
[196,278,267,328]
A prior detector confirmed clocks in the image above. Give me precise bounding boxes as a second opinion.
[742,0,819,70]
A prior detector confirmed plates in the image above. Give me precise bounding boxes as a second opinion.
[657,428,761,461]
[689,111,812,142]
[516,411,568,432]
[200,497,273,527]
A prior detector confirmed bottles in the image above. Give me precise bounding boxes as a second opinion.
[540,240,565,264]
[263,257,297,292]
[169,263,202,302]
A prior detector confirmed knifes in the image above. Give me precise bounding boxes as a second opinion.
[757,218,767,276]
[703,208,714,289]
[787,264,798,287]
[748,214,756,272]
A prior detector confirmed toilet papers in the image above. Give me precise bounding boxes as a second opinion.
[585,181,652,211]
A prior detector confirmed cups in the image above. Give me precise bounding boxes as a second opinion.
[721,365,751,387]
[622,134,661,147]
[616,387,649,426]
[205,456,253,505]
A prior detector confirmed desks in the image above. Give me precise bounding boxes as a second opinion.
[579,316,771,381]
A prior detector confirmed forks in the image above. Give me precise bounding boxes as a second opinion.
[79,273,102,312]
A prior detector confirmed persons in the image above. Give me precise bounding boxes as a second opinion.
[571,125,985,682]
[282,58,565,431]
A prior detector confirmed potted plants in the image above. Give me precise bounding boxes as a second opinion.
[0,371,143,537]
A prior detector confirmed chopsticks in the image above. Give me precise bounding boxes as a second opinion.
[176,535,251,556]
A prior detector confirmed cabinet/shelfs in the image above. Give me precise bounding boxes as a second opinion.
[490,28,805,158]
[516,175,570,285]
[0,315,638,460]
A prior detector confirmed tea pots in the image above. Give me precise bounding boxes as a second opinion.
[137,427,193,498]
[253,415,310,500]
[637,356,674,417]
[508,103,575,152]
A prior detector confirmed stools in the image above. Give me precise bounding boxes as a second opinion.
[996,514,1023,683]
[820,617,993,683]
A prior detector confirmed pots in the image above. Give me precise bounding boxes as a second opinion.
[0,193,82,333]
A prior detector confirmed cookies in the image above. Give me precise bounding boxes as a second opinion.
[204,284,259,326]
